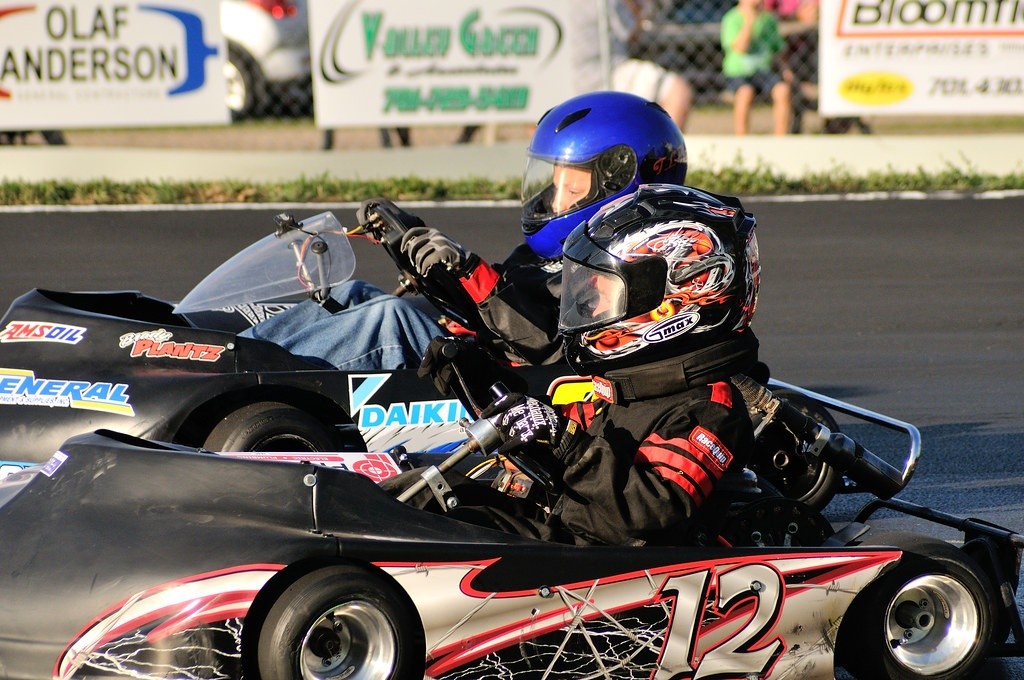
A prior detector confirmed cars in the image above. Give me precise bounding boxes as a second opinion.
[220,0,310,118]
[639,0,818,93]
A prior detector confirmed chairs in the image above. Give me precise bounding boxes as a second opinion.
[742,391,842,511]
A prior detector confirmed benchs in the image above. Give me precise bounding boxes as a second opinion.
[628,35,873,135]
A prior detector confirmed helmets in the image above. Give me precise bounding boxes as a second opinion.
[558,182,760,373]
[521,90,687,258]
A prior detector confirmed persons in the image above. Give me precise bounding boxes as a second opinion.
[608,0,854,134]
[238,92,762,548]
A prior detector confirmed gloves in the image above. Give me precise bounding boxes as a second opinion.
[400,226,477,280]
[419,335,504,399]
[481,390,591,470]
[357,197,412,244]
